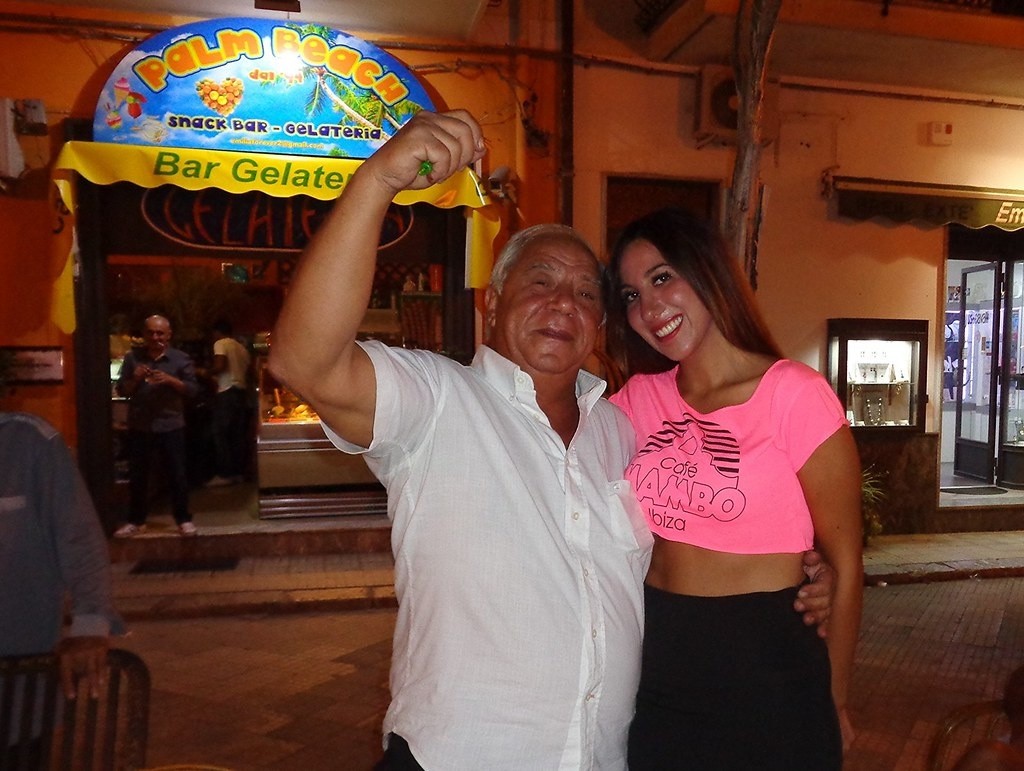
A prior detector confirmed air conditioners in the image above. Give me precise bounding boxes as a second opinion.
[695,63,780,147]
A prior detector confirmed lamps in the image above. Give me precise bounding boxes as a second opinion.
[255,0,300,20]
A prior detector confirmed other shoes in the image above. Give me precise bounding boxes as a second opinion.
[177,521,198,537]
[112,522,148,539]
[205,472,243,488]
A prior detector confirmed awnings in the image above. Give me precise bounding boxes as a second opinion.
[45,140,502,335]
[823,172,1024,232]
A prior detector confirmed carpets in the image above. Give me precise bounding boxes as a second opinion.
[941,487,1009,495]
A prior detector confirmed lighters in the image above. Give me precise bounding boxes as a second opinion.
[419,160,432,176]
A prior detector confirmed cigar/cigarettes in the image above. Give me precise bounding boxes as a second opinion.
[159,342,164,346]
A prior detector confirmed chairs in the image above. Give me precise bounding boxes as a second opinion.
[0,647,154,770]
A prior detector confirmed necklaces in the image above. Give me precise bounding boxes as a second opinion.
[867,395,882,426]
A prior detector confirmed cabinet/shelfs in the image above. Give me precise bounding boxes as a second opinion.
[822,314,932,427]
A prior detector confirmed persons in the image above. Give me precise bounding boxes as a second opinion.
[605,204,864,771]
[269,109,837,771]
[0,412,112,771]
[115,315,199,538]
[196,321,252,486]
